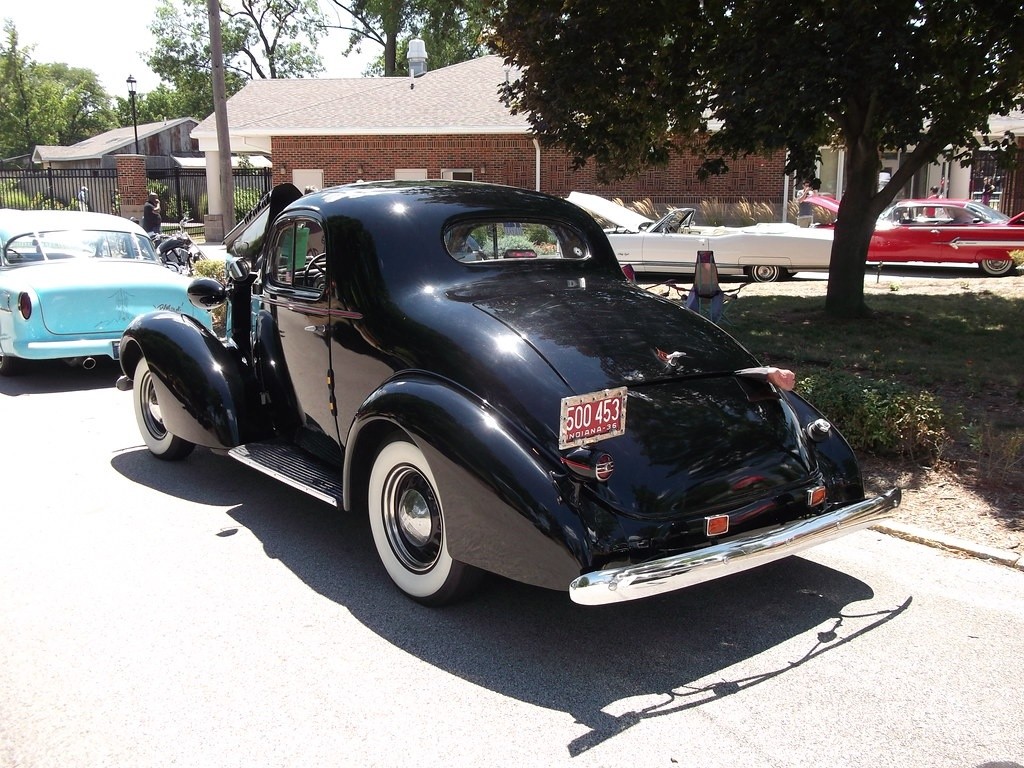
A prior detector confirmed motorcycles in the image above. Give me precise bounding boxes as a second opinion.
[129,211,212,278]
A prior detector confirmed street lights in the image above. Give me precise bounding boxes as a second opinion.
[124,74,141,155]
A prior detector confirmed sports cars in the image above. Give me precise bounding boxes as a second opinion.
[568,186,835,282]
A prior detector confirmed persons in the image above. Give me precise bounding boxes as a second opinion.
[143,191,161,235]
[798,182,813,228]
[923,178,995,218]
[77,187,88,210]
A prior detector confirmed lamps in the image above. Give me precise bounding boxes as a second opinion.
[357,163,364,175]
[280,163,286,175]
[482,162,486,175]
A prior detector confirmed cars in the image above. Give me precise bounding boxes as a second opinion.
[114,179,906,611]
[803,193,1024,278]
[0,208,215,377]
[444,228,485,262]
